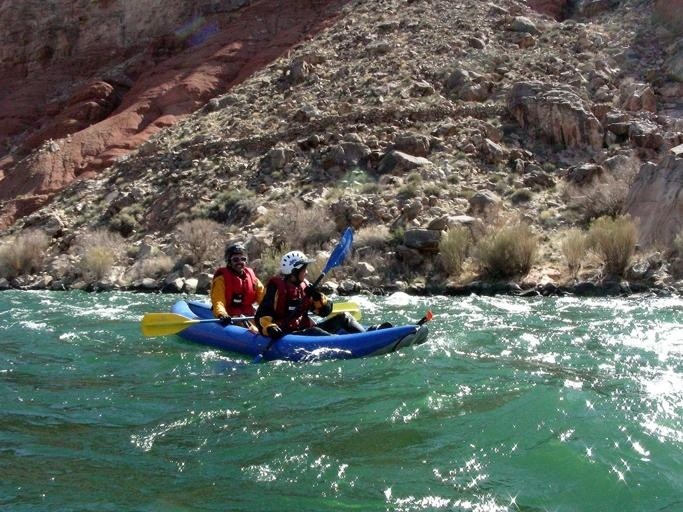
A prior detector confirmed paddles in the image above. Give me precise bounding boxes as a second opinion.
[142,303,362,337]
[252,228,353,363]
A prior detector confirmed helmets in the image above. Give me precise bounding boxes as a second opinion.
[281,250,317,274]
[224,243,246,262]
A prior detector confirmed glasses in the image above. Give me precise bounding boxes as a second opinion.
[231,257,246,262]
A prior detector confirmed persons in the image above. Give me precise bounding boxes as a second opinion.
[207,241,266,332]
[254,249,390,339]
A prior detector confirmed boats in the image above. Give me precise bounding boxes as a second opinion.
[164,299,429,364]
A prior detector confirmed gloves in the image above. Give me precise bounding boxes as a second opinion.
[310,284,321,301]
[268,326,285,340]
[220,315,231,326]
